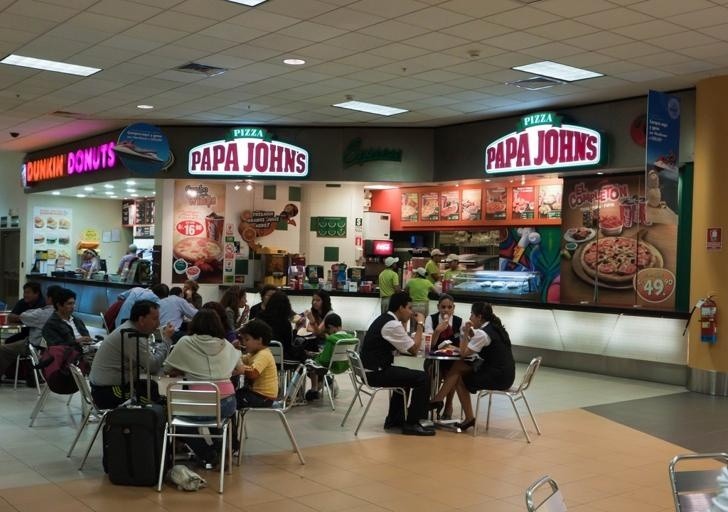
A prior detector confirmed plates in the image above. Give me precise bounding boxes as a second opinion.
[297,330,312,337]
[565,227,597,244]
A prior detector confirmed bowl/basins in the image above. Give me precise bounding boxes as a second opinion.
[598,222,624,236]
[174,258,189,275]
[185,266,201,281]
[565,242,578,254]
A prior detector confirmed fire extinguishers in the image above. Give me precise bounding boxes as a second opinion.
[682,294,718,343]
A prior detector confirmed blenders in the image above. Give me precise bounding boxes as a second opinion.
[29,249,49,276]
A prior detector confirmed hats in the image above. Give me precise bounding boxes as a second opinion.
[384,256,399,268]
[431,248,446,257]
[129,244,138,251]
[83,248,98,256]
[441,253,459,263]
[412,267,427,278]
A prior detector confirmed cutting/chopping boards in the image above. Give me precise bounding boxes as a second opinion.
[569,228,665,290]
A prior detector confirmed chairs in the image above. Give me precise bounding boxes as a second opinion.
[525,475,569,512]
[668,451,728,512]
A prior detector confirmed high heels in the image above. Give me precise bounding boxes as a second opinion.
[460,417,476,432]
[423,399,445,413]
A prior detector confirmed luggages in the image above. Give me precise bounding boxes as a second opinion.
[103,403,170,487]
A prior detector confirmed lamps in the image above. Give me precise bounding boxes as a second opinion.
[185,184,209,201]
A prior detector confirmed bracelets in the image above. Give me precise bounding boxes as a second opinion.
[418,322,424,327]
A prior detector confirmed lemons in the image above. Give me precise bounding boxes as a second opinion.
[238,209,256,242]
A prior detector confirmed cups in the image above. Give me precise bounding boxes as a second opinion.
[0,314,8,326]
[128,205,135,224]
[618,199,653,228]
[441,279,450,294]
[204,216,224,243]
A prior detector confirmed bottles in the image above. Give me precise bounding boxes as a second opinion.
[582,209,592,228]
[293,270,338,291]
[591,207,601,231]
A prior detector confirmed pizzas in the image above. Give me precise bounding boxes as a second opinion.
[584,238,655,284]
[175,236,222,265]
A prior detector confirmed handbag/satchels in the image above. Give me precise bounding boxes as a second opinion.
[170,463,209,493]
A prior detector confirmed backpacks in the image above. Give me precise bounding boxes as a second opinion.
[40,345,90,395]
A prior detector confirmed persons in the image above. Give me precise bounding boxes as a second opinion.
[81,249,101,271]
[426,302,515,431]
[1,282,92,387]
[89,281,307,470]
[305,289,355,400]
[424,293,466,419]
[360,292,436,436]
[404,267,438,334]
[425,249,444,284]
[443,254,467,284]
[379,257,400,315]
[117,245,138,274]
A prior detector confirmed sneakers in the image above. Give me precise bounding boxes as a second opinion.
[305,377,339,400]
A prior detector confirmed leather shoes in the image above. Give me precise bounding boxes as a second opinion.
[402,422,434,437]
[442,408,454,420]
[382,416,405,429]
